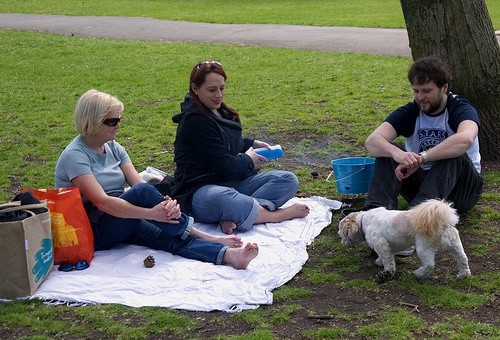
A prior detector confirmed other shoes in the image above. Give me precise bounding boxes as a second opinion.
[398,245,415,256]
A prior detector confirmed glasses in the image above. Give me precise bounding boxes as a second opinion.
[102,118,120,126]
[194,60,223,76]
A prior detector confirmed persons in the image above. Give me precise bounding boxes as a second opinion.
[365,55,484,257]
[55,89,259,270]
[171,61,309,235]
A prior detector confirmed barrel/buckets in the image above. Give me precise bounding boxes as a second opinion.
[326,156,376,194]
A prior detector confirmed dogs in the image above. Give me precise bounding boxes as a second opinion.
[338,197,471,281]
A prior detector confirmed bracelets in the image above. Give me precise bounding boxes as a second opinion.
[419,150,432,170]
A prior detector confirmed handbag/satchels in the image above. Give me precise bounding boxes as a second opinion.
[0,199,53,298]
[22,186,94,265]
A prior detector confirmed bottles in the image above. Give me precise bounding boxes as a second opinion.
[254,144,283,160]
